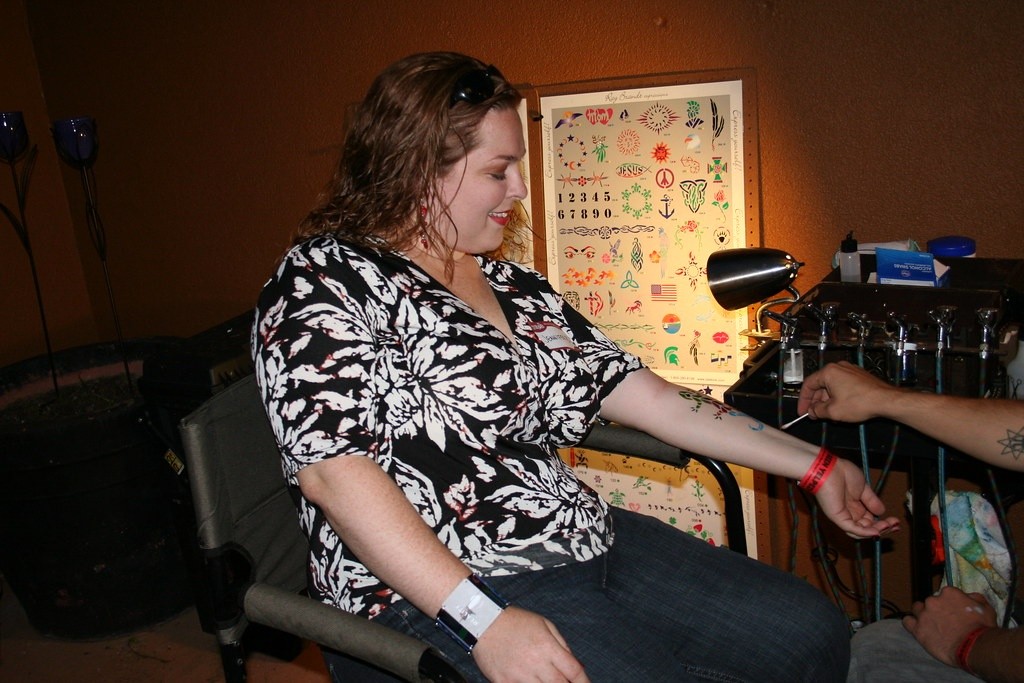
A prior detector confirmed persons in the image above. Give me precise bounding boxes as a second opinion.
[796,360,1024,683]
[250,50,904,683]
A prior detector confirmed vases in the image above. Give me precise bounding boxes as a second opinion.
[0,333,191,643]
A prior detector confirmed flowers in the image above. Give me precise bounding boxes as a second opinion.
[0,110,138,404]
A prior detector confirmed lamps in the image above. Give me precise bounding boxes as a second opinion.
[707,248,807,352]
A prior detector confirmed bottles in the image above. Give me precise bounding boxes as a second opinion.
[1007,324,1024,401]
[840,231,861,282]
[783,321,803,385]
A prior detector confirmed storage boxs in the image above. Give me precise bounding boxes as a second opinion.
[811,256,1024,348]
[875,246,953,289]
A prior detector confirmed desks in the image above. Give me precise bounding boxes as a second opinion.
[723,341,1024,618]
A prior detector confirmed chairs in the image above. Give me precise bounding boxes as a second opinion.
[175,371,750,683]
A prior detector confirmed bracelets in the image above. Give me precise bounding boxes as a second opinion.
[798,446,837,495]
[435,572,511,655]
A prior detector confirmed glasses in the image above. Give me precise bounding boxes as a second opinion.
[447,65,510,109]
